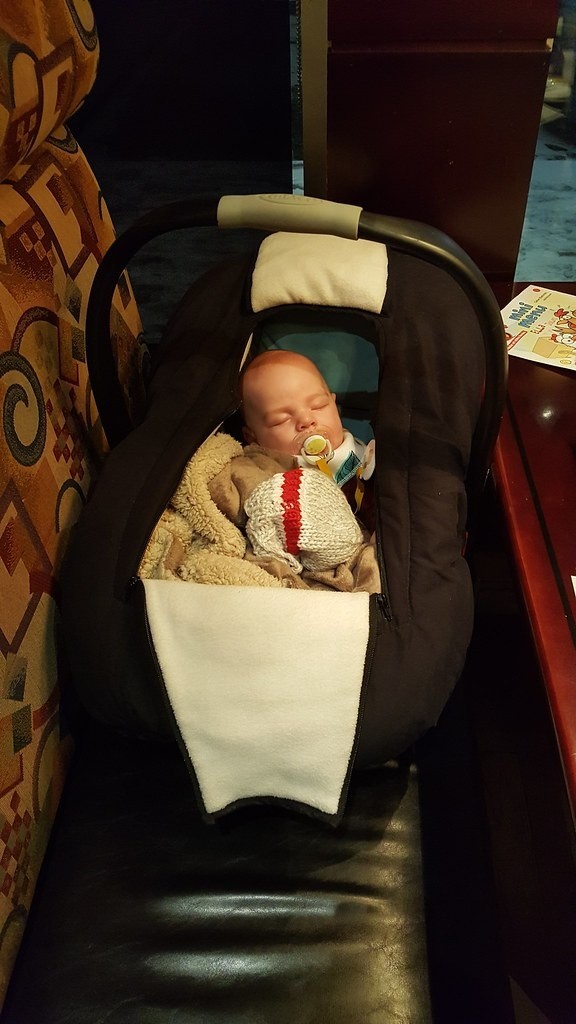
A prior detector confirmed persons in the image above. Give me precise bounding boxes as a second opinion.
[236,350,343,456]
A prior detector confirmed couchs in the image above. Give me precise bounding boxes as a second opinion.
[0,0,433,1024]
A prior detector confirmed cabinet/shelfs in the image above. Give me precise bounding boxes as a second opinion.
[300,0,576,280]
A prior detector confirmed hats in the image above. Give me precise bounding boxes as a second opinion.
[243,468,363,574]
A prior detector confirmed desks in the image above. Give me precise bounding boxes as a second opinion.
[467,279,576,1024]
